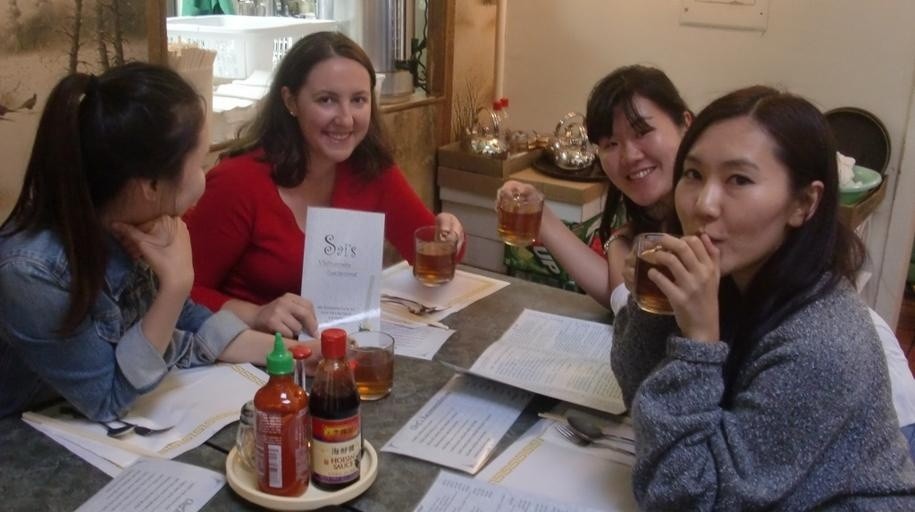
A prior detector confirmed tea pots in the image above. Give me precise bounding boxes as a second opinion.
[543,111,599,171]
[273,0,317,18]
[461,107,509,163]
[231,0,267,16]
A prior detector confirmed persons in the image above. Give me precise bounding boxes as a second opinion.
[1,60,357,423]
[181,29,467,342]
[608,84,915,512]
[494,63,698,319]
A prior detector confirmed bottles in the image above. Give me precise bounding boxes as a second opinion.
[233,399,257,471]
[286,342,309,395]
[308,328,361,492]
[254,332,310,498]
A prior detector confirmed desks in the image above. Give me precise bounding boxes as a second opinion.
[2,267,638,511]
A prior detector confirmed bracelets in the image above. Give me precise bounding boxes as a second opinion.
[603,232,629,254]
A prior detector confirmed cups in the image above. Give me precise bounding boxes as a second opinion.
[342,329,394,403]
[505,122,550,153]
[411,225,459,288]
[495,187,546,249]
[631,232,676,315]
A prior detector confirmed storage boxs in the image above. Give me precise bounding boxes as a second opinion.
[434,163,605,289]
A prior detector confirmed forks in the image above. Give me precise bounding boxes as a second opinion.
[554,423,636,457]
[379,293,454,312]
[117,418,175,437]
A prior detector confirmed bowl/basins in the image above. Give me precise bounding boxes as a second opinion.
[837,164,885,207]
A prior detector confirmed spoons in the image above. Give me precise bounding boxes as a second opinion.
[379,299,438,315]
[566,413,636,443]
[58,406,137,440]
